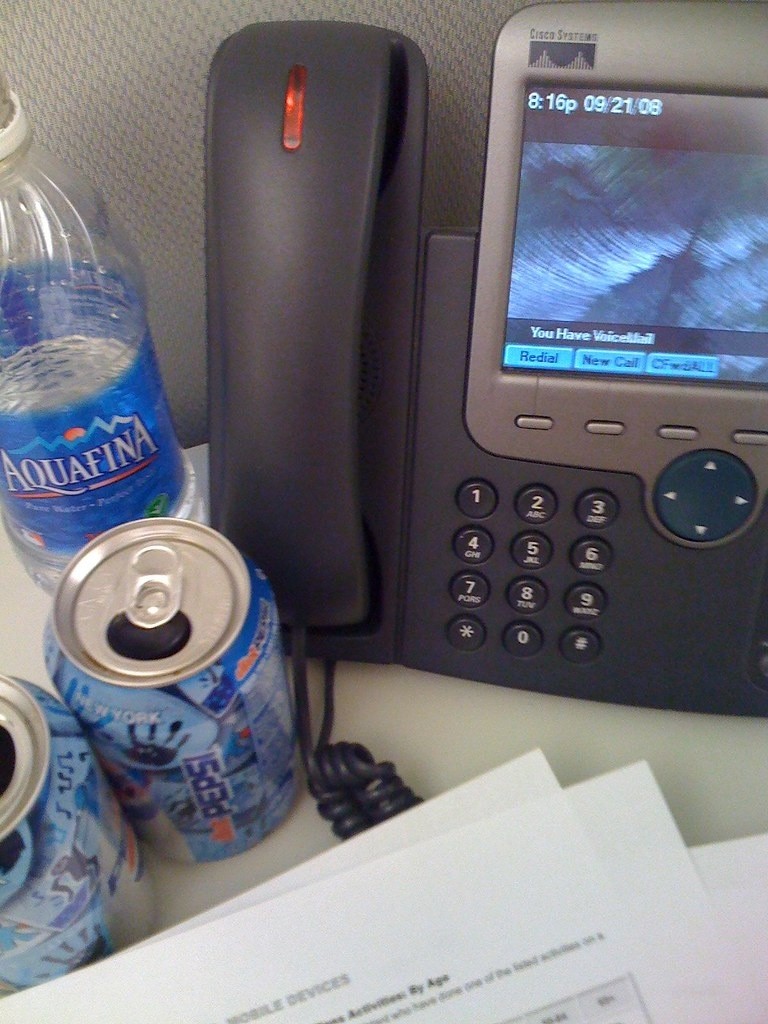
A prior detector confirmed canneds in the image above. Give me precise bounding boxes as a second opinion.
[0,674,156,990]
[39,518,307,866]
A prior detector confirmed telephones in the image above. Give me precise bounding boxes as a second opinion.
[203,0,768,719]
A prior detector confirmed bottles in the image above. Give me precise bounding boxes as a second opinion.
[0,65,208,600]
